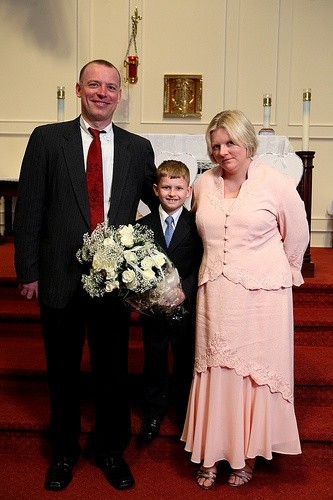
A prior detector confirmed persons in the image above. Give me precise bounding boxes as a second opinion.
[190,108,311,491]
[118,159,203,449]
[12,60,163,492]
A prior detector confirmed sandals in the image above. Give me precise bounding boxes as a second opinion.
[228,463,254,487]
[196,463,218,489]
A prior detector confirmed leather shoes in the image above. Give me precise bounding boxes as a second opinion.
[95,451,135,490]
[44,446,78,491]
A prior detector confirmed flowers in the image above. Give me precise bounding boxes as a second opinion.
[75,221,166,298]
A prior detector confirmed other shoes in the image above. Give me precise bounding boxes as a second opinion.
[140,407,165,443]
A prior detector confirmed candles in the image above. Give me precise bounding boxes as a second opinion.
[128,55,139,85]
[56,86,65,122]
[258,88,312,151]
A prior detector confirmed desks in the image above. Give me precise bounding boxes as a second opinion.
[136,133,294,278]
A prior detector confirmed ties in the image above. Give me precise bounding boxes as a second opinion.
[164,216,175,248]
[85,127,106,236]
